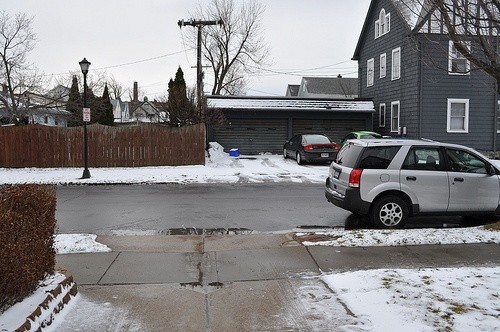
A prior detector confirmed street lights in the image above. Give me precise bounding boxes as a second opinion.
[78,57,92,180]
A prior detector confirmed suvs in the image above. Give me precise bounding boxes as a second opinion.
[323,138,500,229]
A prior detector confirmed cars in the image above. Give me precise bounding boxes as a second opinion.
[283,133,340,164]
[339,130,383,149]
